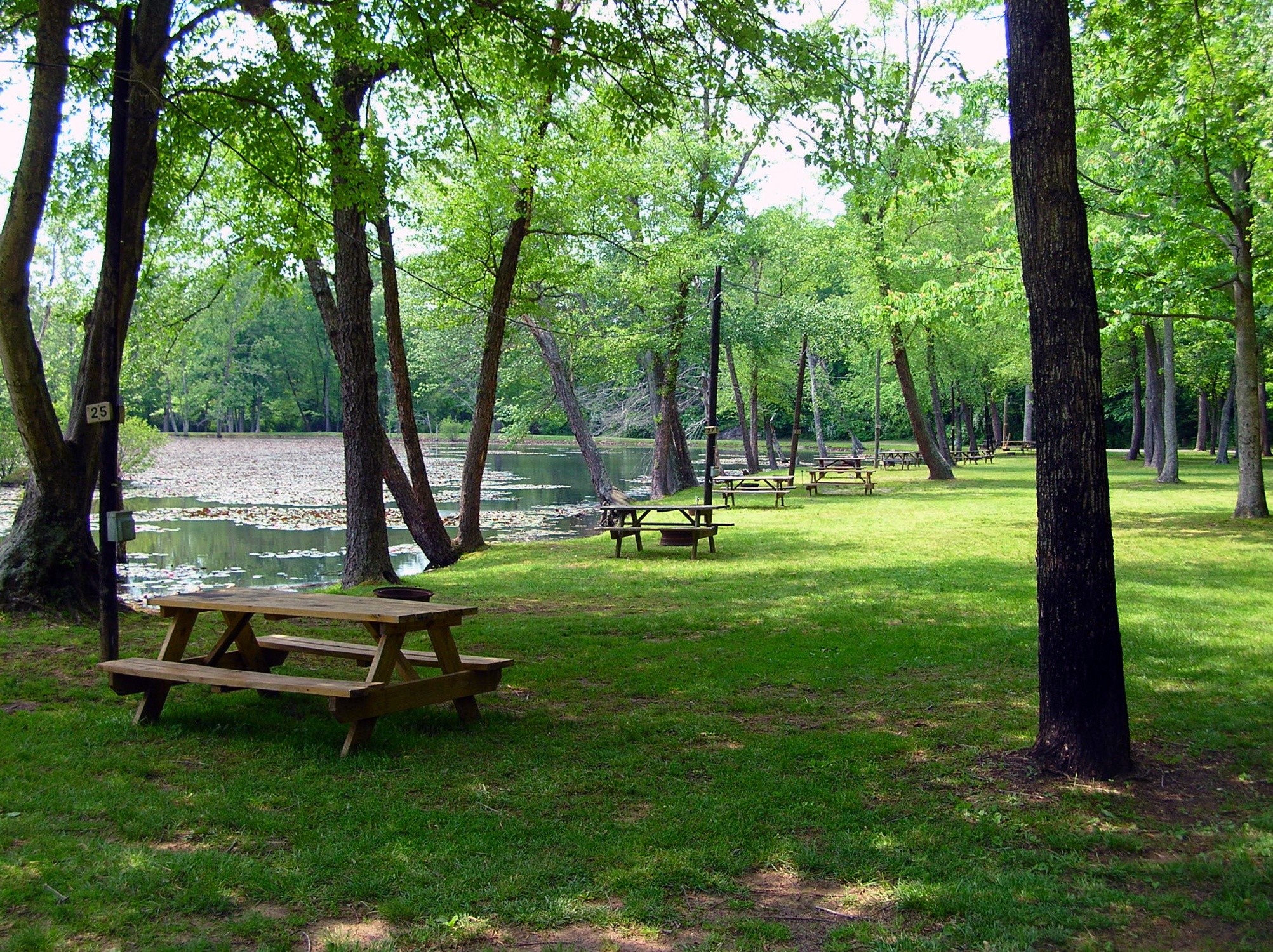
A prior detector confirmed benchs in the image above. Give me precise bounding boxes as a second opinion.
[804,445,1030,489]
[712,485,796,499]
[595,520,735,538]
[96,635,513,719]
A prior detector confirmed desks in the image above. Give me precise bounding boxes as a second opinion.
[713,476,795,507]
[910,452,923,467]
[806,470,876,496]
[878,451,911,470]
[967,449,992,465]
[1001,440,1031,452]
[600,503,730,556]
[813,457,865,478]
[129,587,480,756]
[951,449,967,465]
